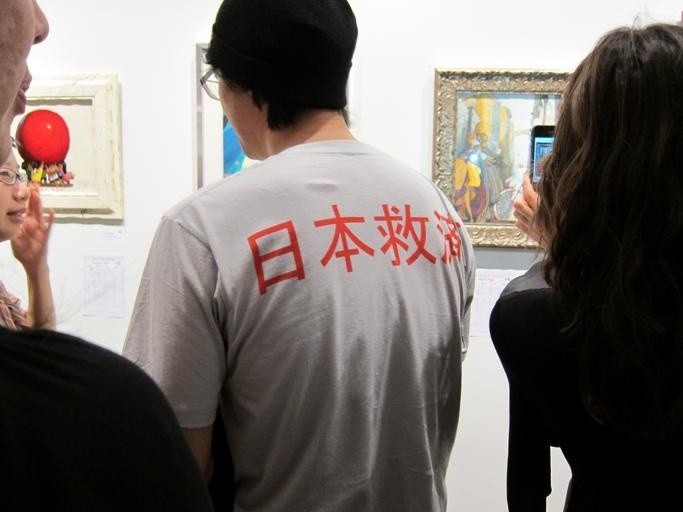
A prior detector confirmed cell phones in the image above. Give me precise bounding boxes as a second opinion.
[529,125,557,191]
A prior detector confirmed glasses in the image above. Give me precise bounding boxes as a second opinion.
[0,167,28,186]
[197,64,225,103]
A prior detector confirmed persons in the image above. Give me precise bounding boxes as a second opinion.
[0,136,56,330]
[123,0,475,512]
[0,0,210,512]
[489,24,683,512]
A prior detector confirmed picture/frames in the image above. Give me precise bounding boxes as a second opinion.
[192,42,271,192]
[14,79,129,224]
[432,67,571,253]
